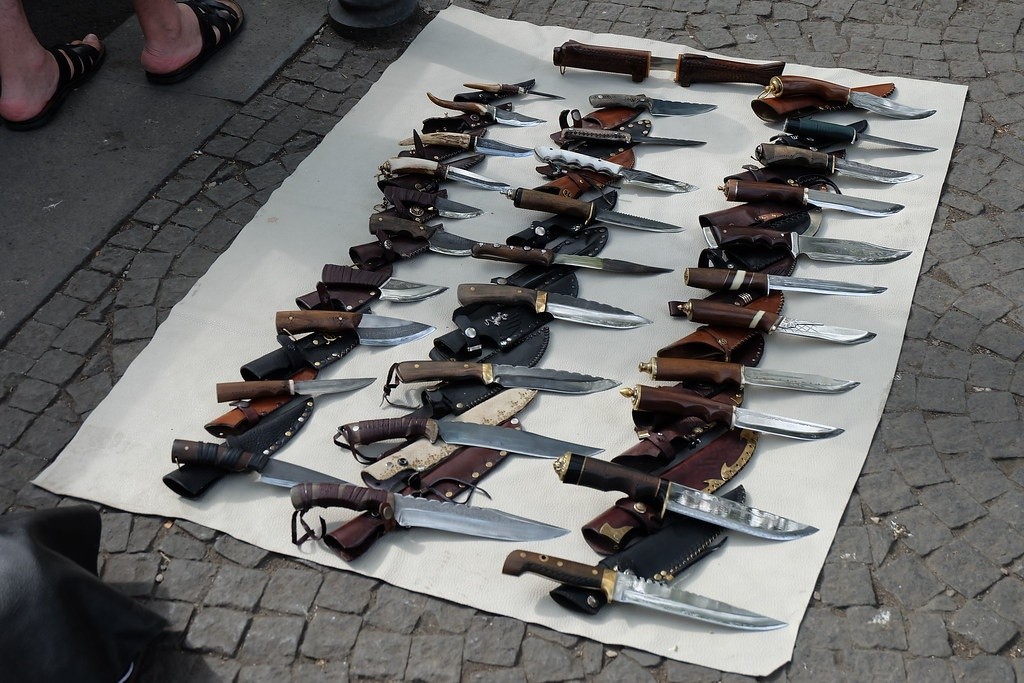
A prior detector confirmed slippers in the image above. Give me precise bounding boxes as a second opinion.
[3,40,106,130]
[144,0,245,84]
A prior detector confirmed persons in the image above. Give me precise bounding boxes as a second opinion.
[0,0,244,129]
